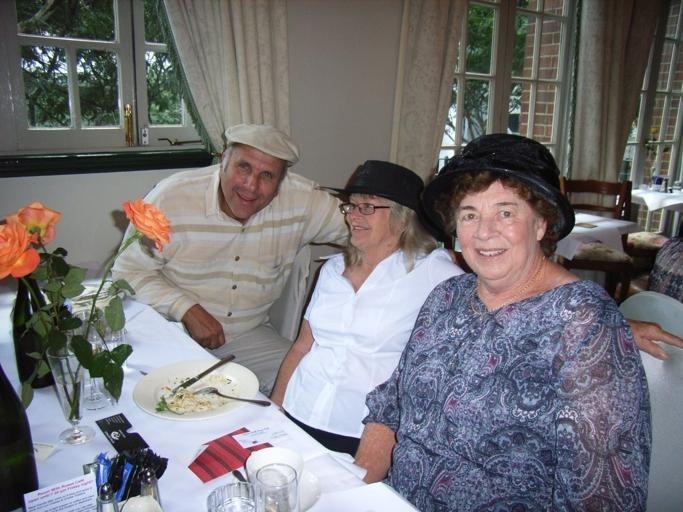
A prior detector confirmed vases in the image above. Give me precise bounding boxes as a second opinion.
[46,345,97,445]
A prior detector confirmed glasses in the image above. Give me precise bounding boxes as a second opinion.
[337,200,398,214]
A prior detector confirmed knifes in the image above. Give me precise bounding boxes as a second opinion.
[166,354,236,393]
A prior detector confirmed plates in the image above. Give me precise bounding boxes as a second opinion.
[246,465,321,512]
[128,361,261,422]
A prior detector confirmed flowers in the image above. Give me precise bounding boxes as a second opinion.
[0,198,176,421]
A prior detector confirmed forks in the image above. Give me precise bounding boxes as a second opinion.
[190,386,271,407]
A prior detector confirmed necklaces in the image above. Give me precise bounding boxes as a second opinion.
[471,257,545,316]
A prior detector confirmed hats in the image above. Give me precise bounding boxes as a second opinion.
[317,158,429,215]
[223,123,302,166]
[428,132,577,241]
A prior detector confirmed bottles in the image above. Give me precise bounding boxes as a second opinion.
[94,469,160,512]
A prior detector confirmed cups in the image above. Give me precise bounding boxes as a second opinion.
[206,462,300,511]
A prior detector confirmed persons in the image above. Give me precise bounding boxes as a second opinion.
[354,134,652,511]
[268,159,683,461]
[109,123,459,399]
